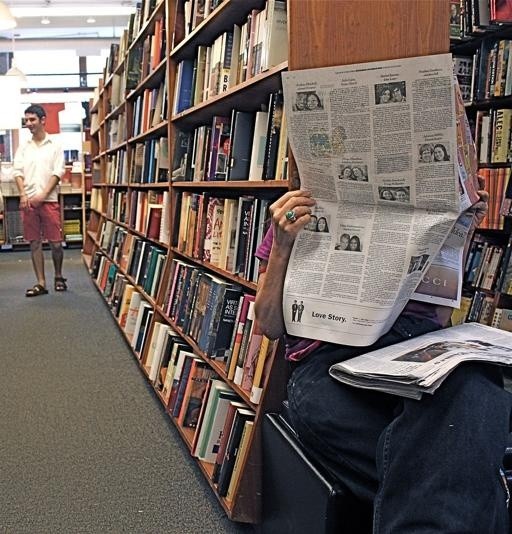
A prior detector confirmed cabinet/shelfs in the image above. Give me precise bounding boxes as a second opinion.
[81,0,450,525]
[0,192,83,252]
[81,119,93,249]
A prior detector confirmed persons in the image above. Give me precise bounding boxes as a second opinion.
[304,215,331,233]
[291,300,304,323]
[13,105,67,297]
[254,172,512,534]
[378,83,407,104]
[339,167,368,181]
[335,234,362,252]
[296,93,322,110]
[380,188,409,203]
[419,143,449,162]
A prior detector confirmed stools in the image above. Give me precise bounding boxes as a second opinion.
[261,400,375,534]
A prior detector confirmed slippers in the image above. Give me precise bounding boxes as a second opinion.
[54,277,67,291]
[26,284,48,297]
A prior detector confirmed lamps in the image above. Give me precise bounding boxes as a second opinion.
[5,28,25,76]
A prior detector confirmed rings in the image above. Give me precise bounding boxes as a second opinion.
[285,211,296,222]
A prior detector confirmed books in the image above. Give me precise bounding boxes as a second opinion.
[171,92,287,182]
[447,0,512,332]
[99,220,167,298]
[84,154,92,224]
[91,252,154,360]
[162,259,275,403]
[171,192,274,283]
[107,88,166,149]
[146,322,255,500]
[107,137,168,183]
[105,1,165,114]
[106,188,170,245]
[171,0,288,115]
